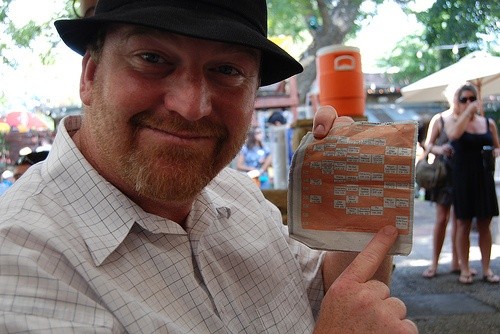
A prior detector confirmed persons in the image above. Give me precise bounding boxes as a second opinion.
[259,111,293,185]
[0,1,418,334]
[422,84,461,277]
[235,125,273,190]
[13,151,48,180]
[445,86,500,283]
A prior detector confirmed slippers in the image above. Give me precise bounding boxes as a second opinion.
[452,268,477,276]
[459,275,473,284]
[482,274,499,283]
[422,269,439,279]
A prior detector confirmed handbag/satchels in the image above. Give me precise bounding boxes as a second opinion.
[415,158,445,189]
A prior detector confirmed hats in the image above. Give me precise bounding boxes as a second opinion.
[54,0,305,86]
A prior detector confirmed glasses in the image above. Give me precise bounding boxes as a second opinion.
[15,155,35,166]
[457,96,478,103]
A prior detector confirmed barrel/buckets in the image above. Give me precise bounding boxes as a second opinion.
[315,46,365,117]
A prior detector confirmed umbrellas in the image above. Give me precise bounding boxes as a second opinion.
[400,49,500,117]
